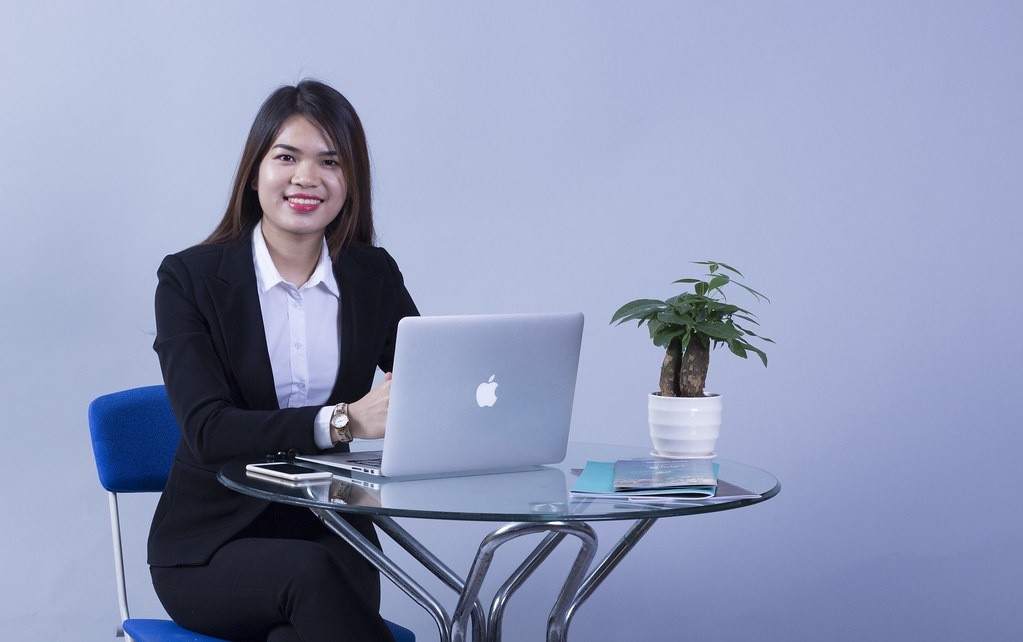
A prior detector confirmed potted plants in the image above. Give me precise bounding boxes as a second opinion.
[606,258,776,462]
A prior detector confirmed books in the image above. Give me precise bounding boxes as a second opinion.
[570,457,763,504]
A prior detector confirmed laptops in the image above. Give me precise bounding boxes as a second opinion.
[294,312,585,477]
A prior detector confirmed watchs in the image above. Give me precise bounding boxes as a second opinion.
[331,402,352,443]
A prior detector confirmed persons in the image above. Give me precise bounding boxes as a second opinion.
[147,79,421,642]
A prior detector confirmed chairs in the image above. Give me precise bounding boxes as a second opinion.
[87,382,418,641]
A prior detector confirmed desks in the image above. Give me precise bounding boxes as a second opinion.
[215,441,780,641]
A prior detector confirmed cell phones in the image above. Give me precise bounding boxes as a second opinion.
[246,462,333,481]
